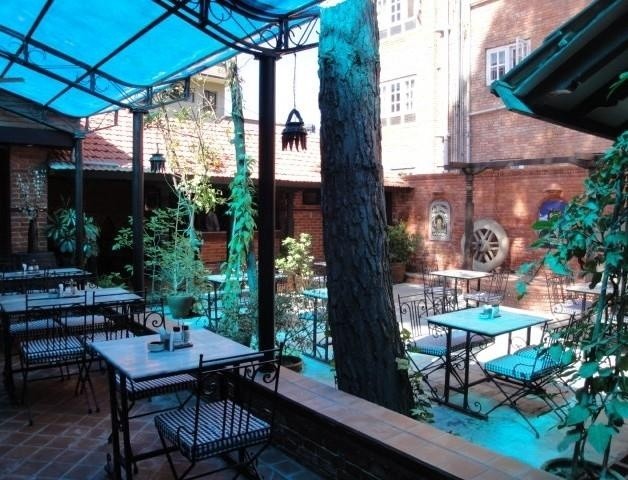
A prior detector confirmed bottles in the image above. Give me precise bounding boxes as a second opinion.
[173,325,190,345]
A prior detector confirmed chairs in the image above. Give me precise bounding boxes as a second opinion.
[154,342,283,480]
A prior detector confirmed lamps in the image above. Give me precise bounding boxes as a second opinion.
[280,51,308,151]
[149,105,167,173]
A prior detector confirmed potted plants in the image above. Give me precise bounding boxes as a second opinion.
[385,218,422,283]
[514,130,628,480]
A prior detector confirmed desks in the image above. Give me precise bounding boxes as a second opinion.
[0,265,262,480]
[203,262,623,420]
